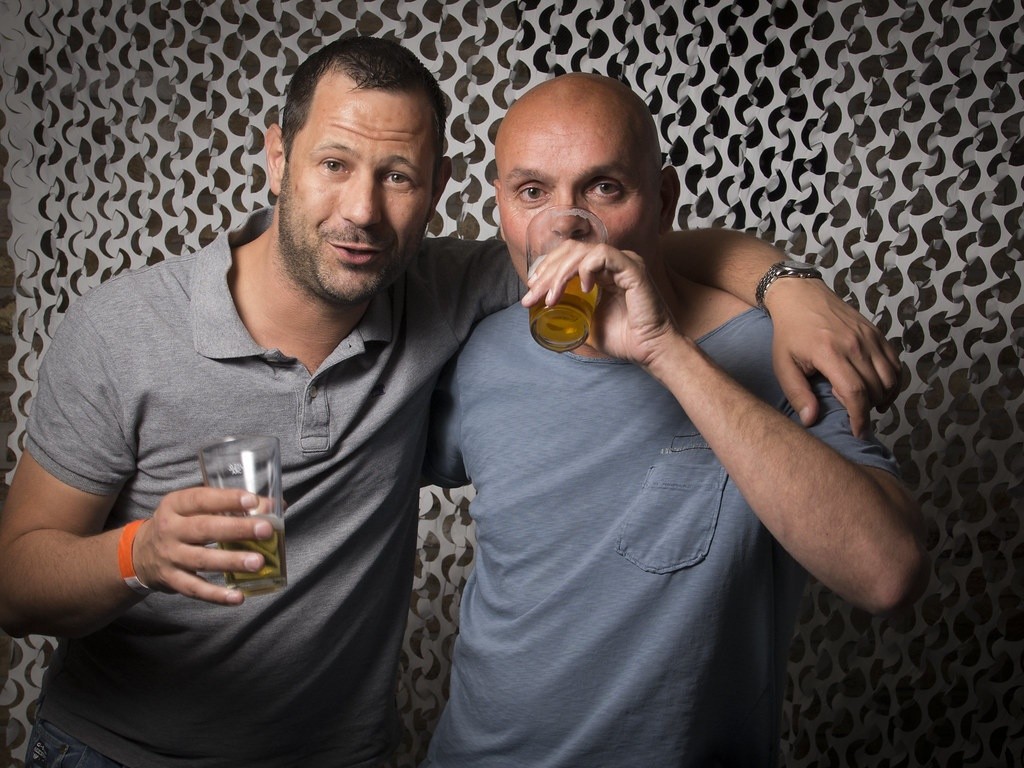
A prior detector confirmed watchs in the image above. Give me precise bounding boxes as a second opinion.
[754,258,825,320]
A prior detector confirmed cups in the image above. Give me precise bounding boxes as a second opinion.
[195,431,287,601]
[524,206,611,354]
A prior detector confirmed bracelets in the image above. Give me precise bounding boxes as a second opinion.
[117,518,159,597]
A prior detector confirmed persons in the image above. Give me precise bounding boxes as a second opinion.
[0,41,916,768]
[407,73,928,768]
